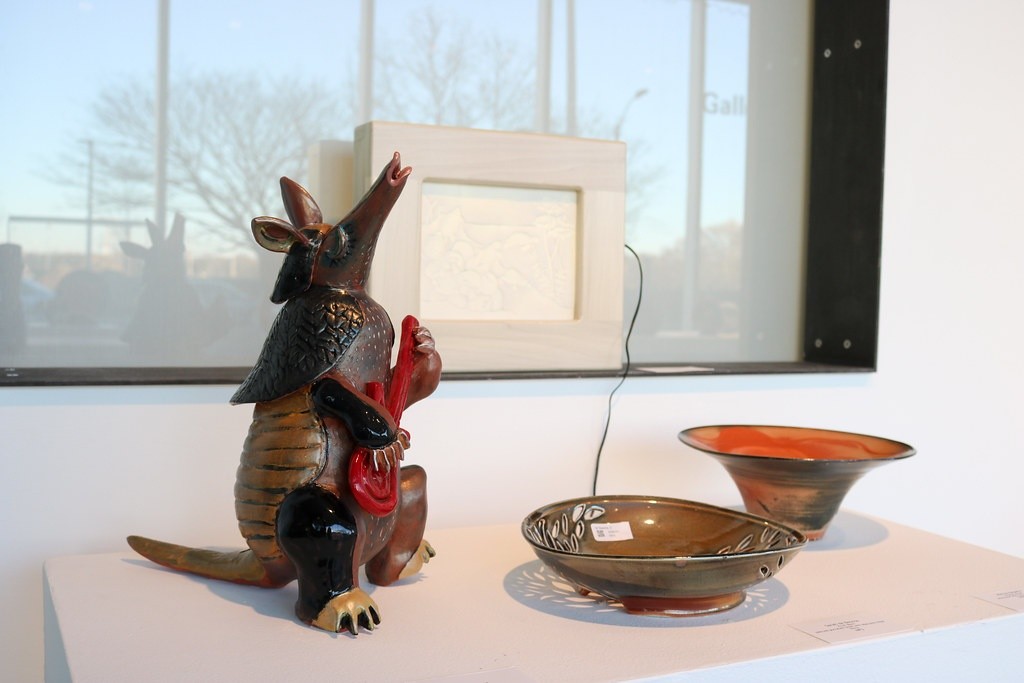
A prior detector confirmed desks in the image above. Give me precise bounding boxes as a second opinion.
[41,503,1024,683]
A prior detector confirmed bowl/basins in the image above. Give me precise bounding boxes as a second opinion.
[521,495,809,618]
